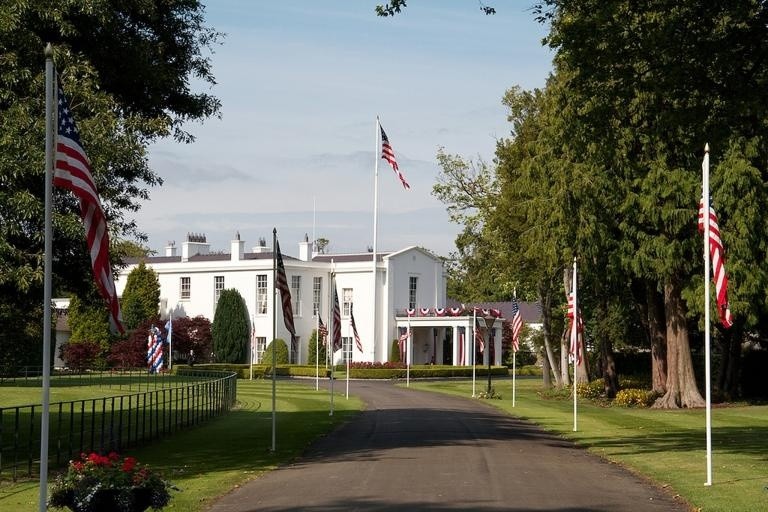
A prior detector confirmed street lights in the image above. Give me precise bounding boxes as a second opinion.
[482,313,495,394]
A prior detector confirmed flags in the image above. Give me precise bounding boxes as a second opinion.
[566,289,583,366]
[697,185,734,328]
[276,240,296,337]
[334,281,342,353]
[380,124,410,190]
[54,70,124,336]
[350,304,365,353]
[319,315,327,345]
[511,296,524,352]
[475,318,485,353]
[164,319,170,344]
